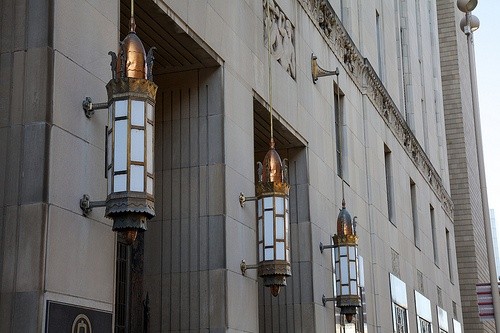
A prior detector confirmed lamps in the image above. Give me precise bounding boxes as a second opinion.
[80,0,158,244]
[310,54,364,323]
[239,1,292,296]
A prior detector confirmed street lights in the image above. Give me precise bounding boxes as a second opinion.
[457,0,500,333]
